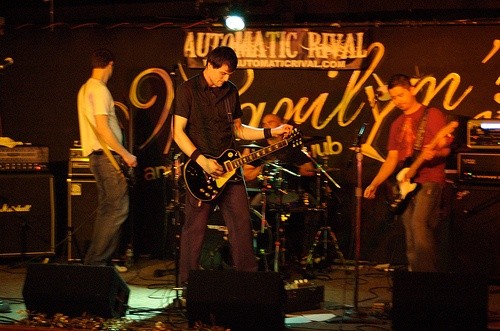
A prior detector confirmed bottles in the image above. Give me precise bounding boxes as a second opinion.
[125,244,134,267]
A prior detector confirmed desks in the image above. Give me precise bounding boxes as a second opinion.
[451,183,500,287]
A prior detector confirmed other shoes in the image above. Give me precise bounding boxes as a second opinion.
[106,262,128,273]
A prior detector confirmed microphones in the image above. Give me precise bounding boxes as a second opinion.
[375,91,383,97]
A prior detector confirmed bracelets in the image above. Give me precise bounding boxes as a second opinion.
[190,149,201,161]
[264,127,272,138]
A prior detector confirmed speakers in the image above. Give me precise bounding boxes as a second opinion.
[22,263,131,320]
[65,178,133,263]
[186,269,287,331]
[0,172,54,257]
[389,272,490,330]
[447,184,500,285]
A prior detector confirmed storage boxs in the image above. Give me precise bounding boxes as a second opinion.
[0,173,56,261]
[67,179,132,263]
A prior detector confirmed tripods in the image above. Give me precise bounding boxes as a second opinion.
[300,142,350,277]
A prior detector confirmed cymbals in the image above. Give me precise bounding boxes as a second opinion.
[308,165,341,175]
[302,136,324,142]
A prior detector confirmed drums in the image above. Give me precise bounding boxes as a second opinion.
[270,174,328,213]
[263,162,298,193]
[194,207,272,272]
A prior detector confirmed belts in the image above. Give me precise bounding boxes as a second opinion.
[93,151,118,155]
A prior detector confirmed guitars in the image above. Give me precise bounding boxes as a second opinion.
[120,123,137,188]
[183,128,302,201]
[386,119,460,213]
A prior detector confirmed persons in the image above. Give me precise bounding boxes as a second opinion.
[169,46,293,299]
[77,48,138,272]
[363,74,456,274]
[243,114,316,262]
